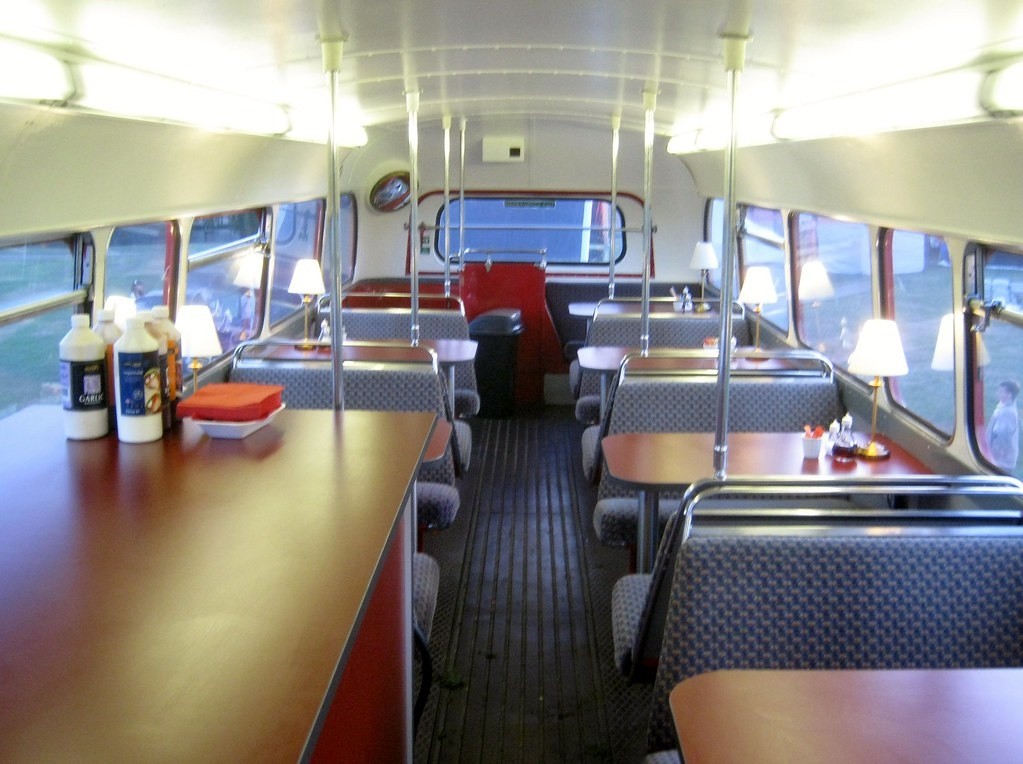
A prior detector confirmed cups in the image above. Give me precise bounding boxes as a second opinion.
[802,433,821,460]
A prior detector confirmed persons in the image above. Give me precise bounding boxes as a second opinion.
[983,381,1019,473]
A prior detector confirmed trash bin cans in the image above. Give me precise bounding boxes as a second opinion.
[467,309,527,418]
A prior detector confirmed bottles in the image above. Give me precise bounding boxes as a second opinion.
[150,305,183,425]
[316,321,334,354]
[702,336,719,349]
[826,420,842,457]
[139,309,175,430]
[57,314,111,440]
[91,308,127,425]
[112,311,166,444]
[833,412,856,463]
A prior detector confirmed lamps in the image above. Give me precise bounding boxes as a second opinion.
[288,259,325,351]
[848,317,909,461]
[739,266,777,351]
[690,240,718,312]
[798,260,836,354]
[232,250,263,341]
[178,304,224,394]
[930,313,991,372]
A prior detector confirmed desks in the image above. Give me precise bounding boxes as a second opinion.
[242,339,479,419]
[577,347,816,430]
[568,301,720,335]
[411,420,453,553]
[668,667,1023,764]
[0,405,440,764]
[600,429,934,575]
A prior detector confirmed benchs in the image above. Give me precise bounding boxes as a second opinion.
[224,280,1022,764]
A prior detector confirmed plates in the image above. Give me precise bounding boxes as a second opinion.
[191,403,286,439]
